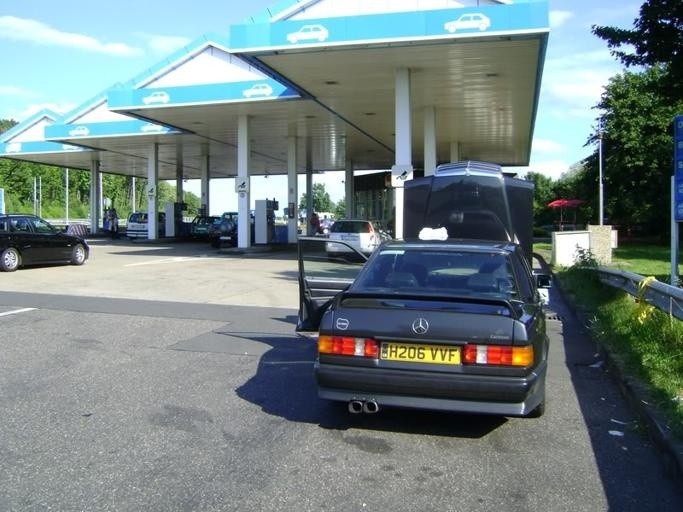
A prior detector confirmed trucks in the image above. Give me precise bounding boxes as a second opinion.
[187,211,255,248]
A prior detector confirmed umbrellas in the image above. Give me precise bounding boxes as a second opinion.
[565,199,588,224]
[547,199,572,224]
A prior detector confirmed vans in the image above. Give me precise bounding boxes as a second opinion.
[125,210,166,241]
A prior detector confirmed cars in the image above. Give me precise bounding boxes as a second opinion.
[0,213,90,273]
[293,158,555,423]
[323,219,382,262]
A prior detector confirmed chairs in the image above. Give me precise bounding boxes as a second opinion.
[16,220,27,231]
[383,261,508,293]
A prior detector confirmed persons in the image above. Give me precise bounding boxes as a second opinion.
[107,208,121,240]
[585,219,591,230]
[603,214,609,225]
[309,213,320,234]
[322,215,331,229]
